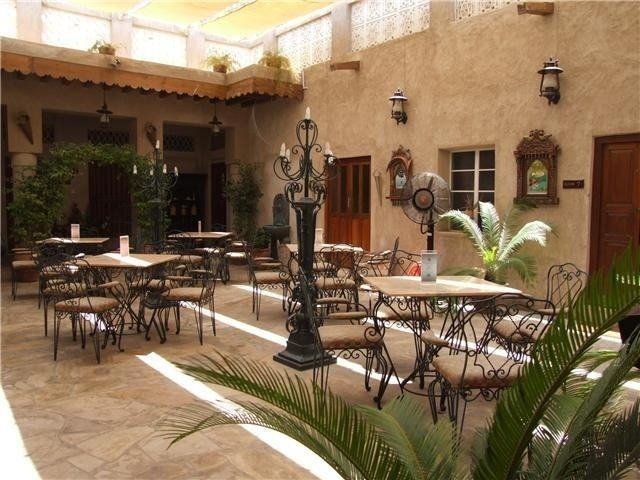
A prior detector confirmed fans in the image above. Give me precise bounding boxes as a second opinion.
[399,171,454,250]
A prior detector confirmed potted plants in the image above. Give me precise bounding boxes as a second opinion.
[5,174,65,284]
[257,47,289,71]
[199,47,242,74]
[92,37,121,55]
[432,197,557,316]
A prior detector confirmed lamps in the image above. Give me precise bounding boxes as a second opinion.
[208,97,223,134]
[536,55,564,107]
[272,104,338,371]
[95,85,114,129]
[133,139,179,279]
[388,88,409,126]
